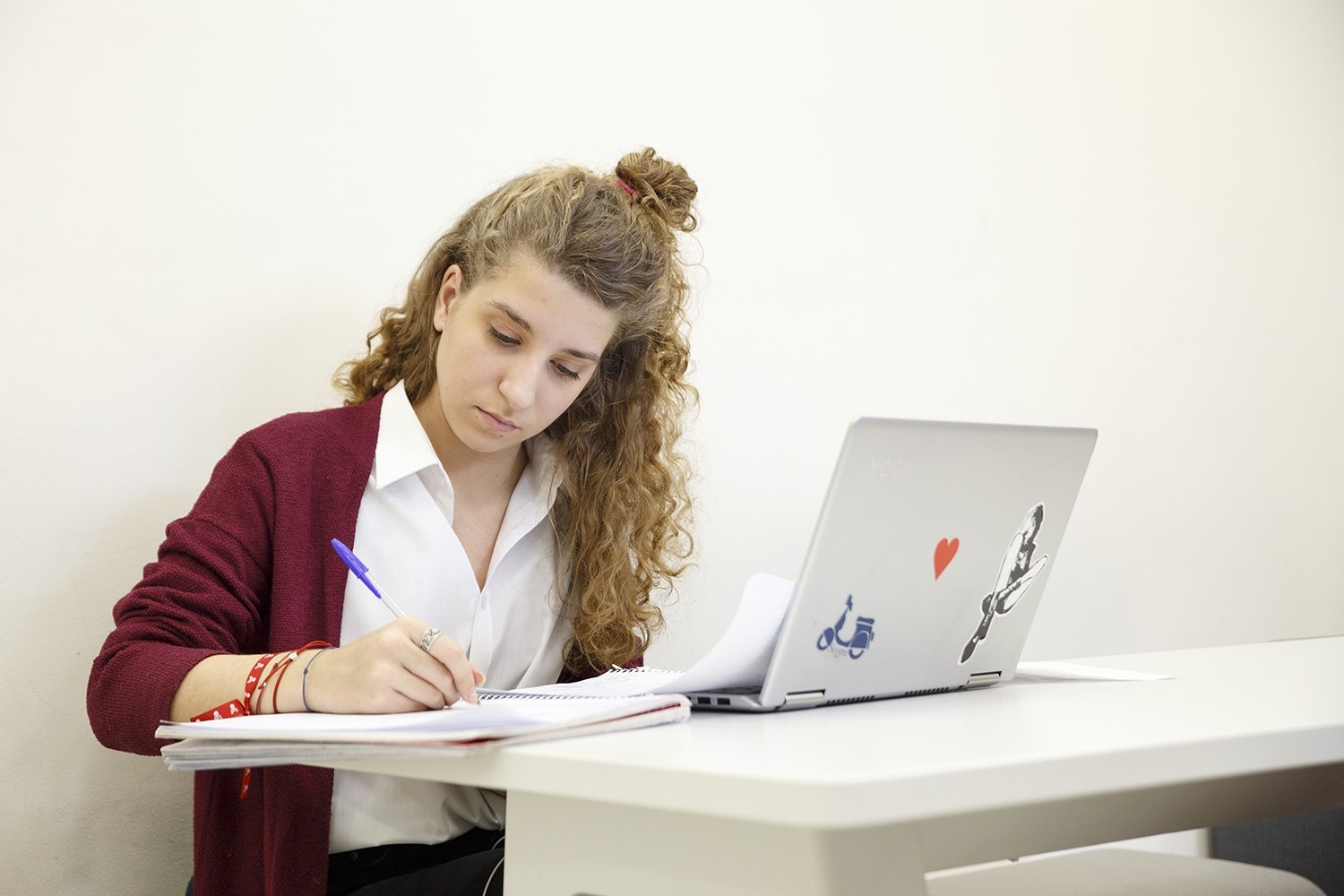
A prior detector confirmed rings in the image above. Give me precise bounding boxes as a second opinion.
[420,626,444,653]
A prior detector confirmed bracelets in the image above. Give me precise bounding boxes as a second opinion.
[189,639,340,724]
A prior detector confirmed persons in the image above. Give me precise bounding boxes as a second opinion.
[82,142,700,896]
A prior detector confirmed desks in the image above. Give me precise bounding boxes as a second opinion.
[292,635,1344,896]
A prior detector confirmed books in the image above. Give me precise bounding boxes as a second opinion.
[145,682,693,775]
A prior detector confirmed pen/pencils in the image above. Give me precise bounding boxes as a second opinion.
[330,537,485,709]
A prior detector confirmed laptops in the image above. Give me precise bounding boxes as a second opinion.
[653,418,1098,719]
